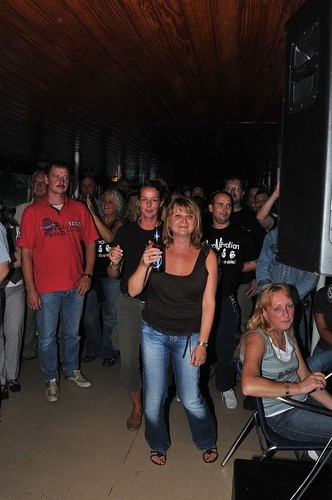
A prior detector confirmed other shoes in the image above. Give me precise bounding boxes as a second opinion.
[8,379,21,392]
[0,386,9,399]
[221,389,237,409]
[127,418,141,431]
[244,396,256,410]
[103,359,116,366]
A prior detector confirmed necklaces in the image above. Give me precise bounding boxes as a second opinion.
[274,333,285,351]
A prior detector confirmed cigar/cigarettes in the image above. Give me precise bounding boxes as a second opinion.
[324,373,332,380]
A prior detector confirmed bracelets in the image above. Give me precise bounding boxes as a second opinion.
[285,381,290,397]
[197,341,208,347]
[110,262,121,269]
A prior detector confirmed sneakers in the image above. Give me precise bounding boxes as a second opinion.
[65,370,91,389]
[46,379,59,402]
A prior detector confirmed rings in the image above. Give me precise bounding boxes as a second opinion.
[111,253,115,257]
[149,255,153,259]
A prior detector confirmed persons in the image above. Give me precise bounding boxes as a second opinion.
[108,180,173,431]
[256,227,319,352]
[305,284,332,373]
[16,161,100,402]
[127,196,218,466]
[0,170,280,409]
[239,284,332,443]
[202,192,257,409]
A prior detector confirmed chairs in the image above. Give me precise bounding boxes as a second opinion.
[220,360,332,467]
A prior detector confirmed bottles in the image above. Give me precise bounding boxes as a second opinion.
[152,227,165,273]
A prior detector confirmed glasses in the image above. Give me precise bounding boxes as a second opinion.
[227,185,240,192]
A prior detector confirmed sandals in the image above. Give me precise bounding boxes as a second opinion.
[150,450,166,466]
[202,447,218,463]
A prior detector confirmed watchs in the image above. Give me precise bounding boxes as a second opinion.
[81,274,93,279]
